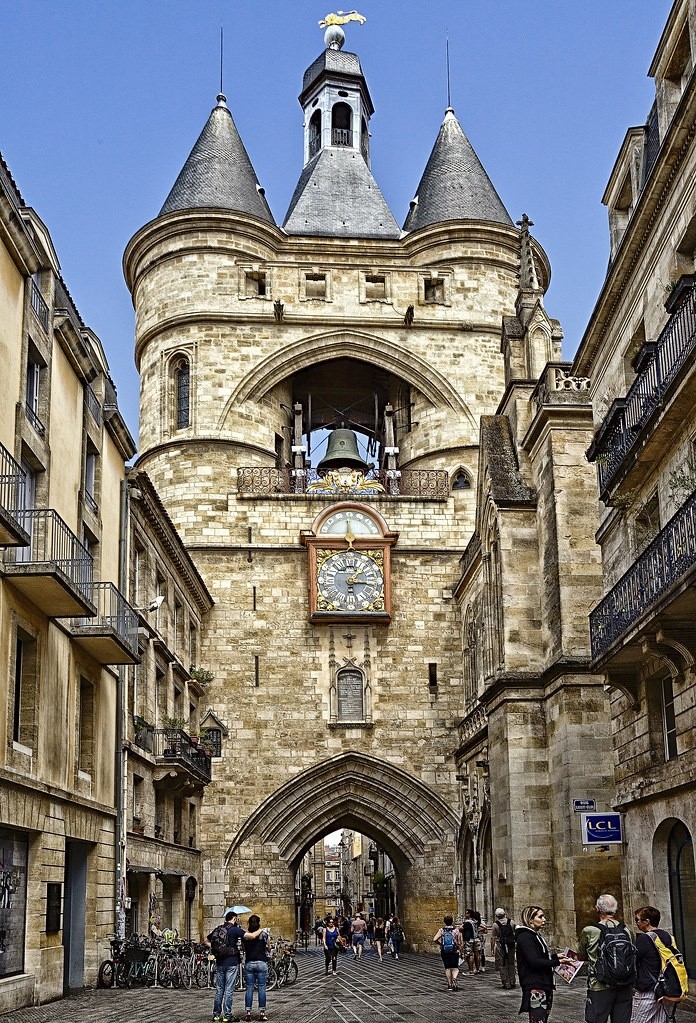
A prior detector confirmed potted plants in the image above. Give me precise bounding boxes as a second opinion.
[134,714,216,758]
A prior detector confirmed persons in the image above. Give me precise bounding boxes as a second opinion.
[234,923,240,928]
[322,918,340,977]
[462,909,487,976]
[208,912,263,1021]
[513,905,576,1023]
[629,906,674,1023]
[577,894,635,1023]
[432,916,463,992]
[241,915,271,1020]
[490,908,518,989]
[315,913,406,962]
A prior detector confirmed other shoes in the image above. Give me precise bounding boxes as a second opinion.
[245,1015,251,1021]
[222,1016,234,1022]
[502,983,515,990]
[213,1016,220,1021]
[260,1015,268,1020]
[462,965,485,976]
[323,946,400,976]
[447,979,458,991]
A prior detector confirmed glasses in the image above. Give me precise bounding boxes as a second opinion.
[635,917,639,922]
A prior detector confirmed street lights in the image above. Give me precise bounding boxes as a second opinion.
[305,887,313,951]
[384,882,389,920]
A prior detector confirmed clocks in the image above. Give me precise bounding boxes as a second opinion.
[299,500,401,625]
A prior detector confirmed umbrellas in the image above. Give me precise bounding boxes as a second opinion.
[223,905,252,917]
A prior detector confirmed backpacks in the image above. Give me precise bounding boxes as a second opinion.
[496,918,515,949]
[592,923,635,987]
[442,928,458,953]
[211,924,238,960]
[647,931,689,1003]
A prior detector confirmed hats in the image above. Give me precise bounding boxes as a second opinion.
[354,912,360,916]
[495,908,505,916]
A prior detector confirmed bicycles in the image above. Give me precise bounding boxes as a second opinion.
[99,928,298,992]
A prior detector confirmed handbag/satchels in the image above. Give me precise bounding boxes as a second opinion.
[402,932,406,941]
[334,942,340,949]
